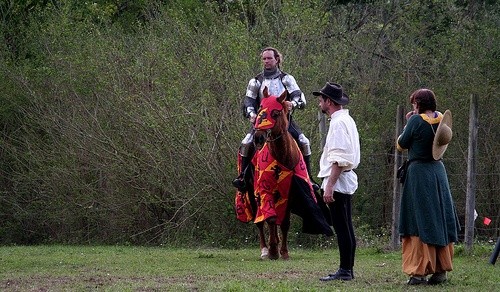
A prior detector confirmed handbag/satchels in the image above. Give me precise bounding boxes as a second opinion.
[397,159,407,184]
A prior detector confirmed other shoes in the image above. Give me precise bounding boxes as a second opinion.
[232,179,242,188]
[427,273,447,285]
[319,269,352,281]
[405,276,428,285]
[328,273,354,279]
[312,182,319,190]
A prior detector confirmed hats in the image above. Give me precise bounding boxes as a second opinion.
[311,82,349,105]
[432,110,452,160]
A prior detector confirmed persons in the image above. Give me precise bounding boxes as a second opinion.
[312,82,361,282]
[397,89,461,286]
[232,47,320,193]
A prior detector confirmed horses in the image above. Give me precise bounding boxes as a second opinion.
[253,86,300,261]
